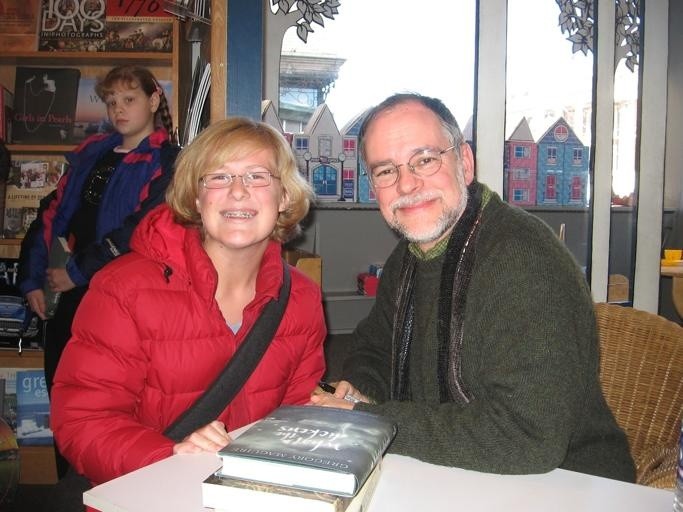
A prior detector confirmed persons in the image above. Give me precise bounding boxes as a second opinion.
[48,115,327,489]
[16,65,183,481]
[308,94,638,487]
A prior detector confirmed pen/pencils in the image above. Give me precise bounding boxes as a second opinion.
[315,377,362,406]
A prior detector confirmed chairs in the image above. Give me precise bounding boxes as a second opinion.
[592,303,681,488]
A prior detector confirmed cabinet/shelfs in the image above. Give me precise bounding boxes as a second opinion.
[0,16,189,487]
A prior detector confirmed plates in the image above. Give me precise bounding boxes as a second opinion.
[661,259,683,266]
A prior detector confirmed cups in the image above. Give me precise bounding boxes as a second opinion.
[664,250,682,261]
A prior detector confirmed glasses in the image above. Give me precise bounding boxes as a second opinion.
[369,144,459,189]
[197,170,282,188]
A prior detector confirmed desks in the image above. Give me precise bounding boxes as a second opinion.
[83,418,677,512]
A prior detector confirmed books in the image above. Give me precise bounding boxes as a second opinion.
[204,403,397,512]
[42,235,72,318]
